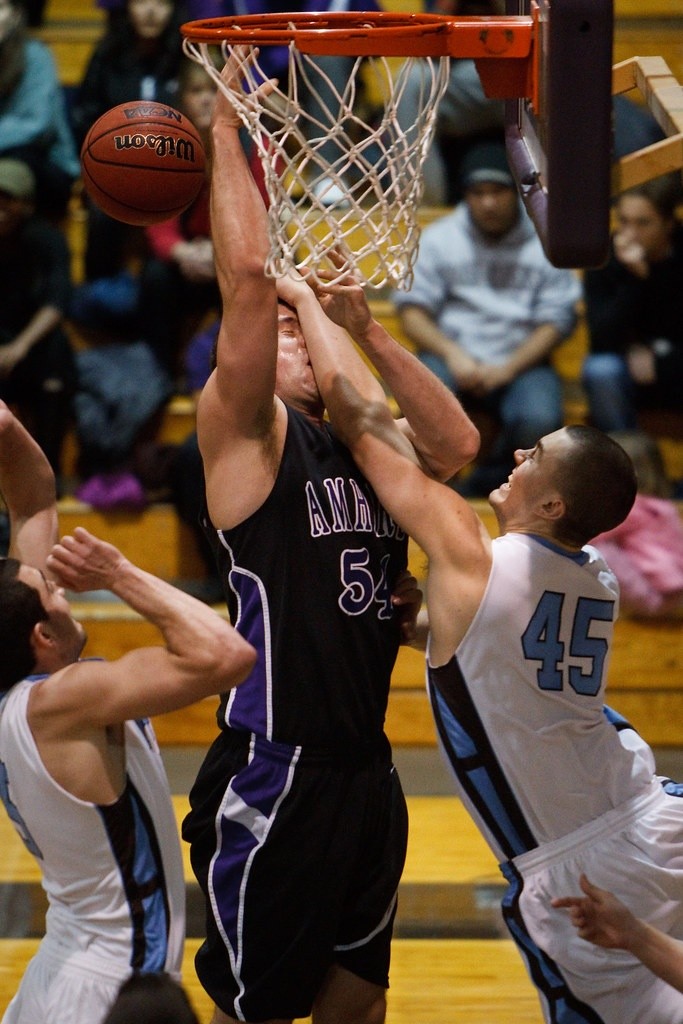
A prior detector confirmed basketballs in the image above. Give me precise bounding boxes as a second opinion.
[78,100,209,227]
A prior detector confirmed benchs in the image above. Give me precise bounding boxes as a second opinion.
[0,0,683,1024]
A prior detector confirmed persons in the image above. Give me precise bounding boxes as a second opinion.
[103,972,203,1024]
[579,167,683,438]
[199,0,384,210]
[551,873,683,994]
[391,1,517,208]
[0,0,86,222]
[51,274,175,489]
[76,0,196,279]
[1,158,80,495]
[0,397,257,1024]
[394,160,586,498]
[274,240,683,1024]
[184,21,481,1024]
[149,56,295,396]
